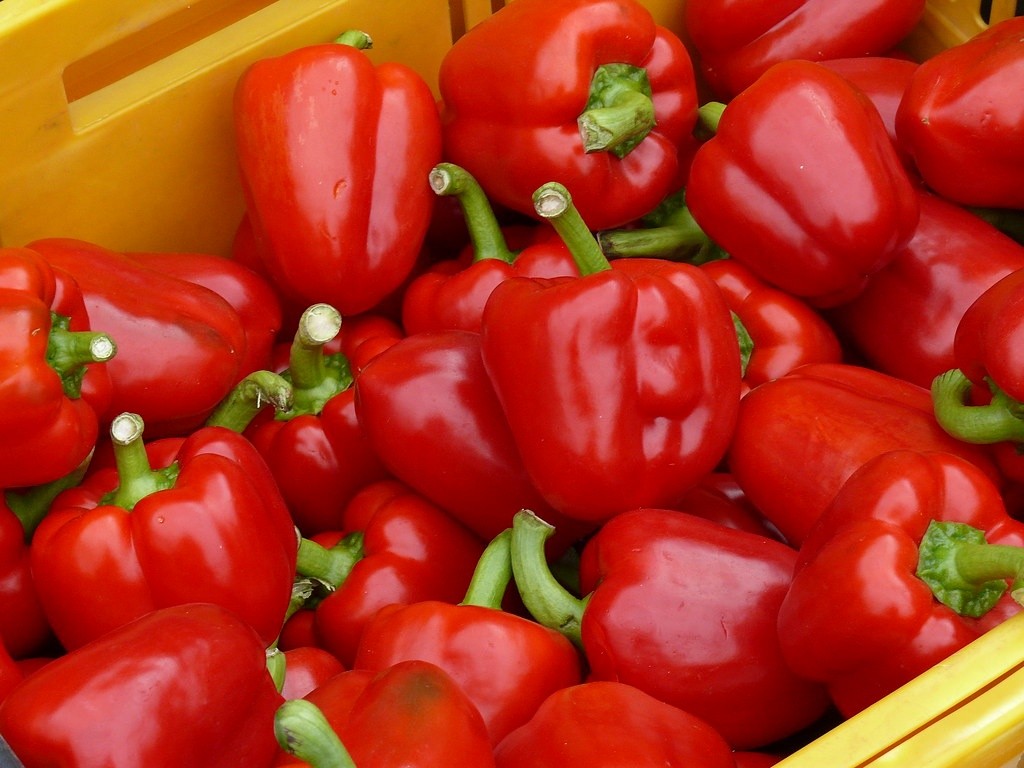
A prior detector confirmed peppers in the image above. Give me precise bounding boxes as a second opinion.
[0,0,1024,768]
